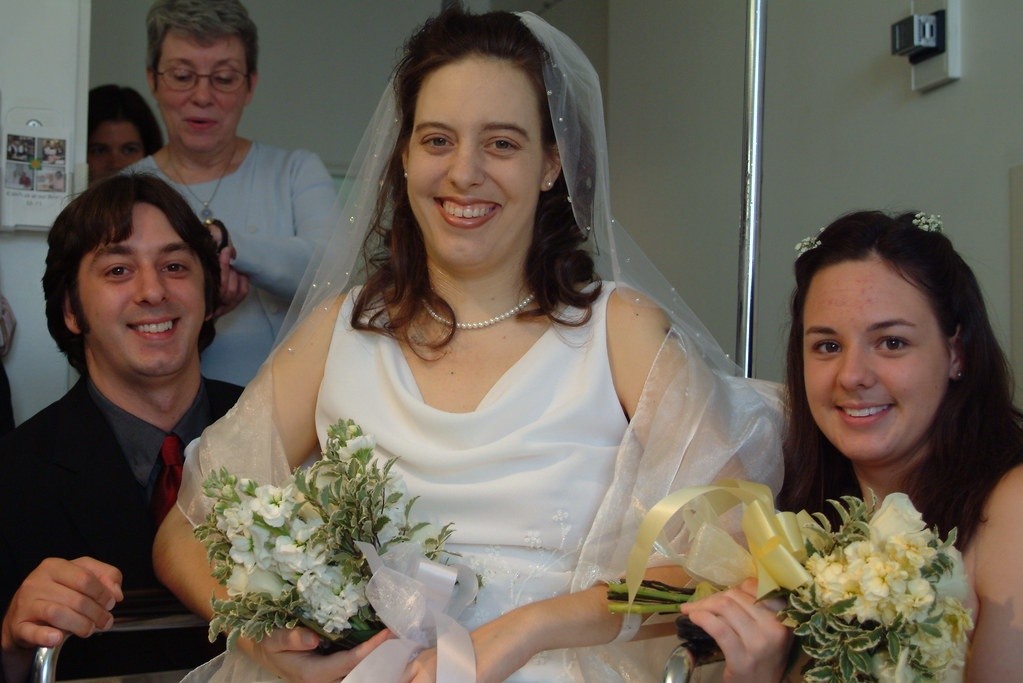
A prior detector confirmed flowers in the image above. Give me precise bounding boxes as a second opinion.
[608,479,975,683]
[190,420,485,649]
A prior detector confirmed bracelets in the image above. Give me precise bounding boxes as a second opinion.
[590,577,643,644]
[205,218,228,253]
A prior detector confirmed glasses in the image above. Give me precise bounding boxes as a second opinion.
[155,67,251,95]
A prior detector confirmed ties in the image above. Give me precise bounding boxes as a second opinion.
[159,432,183,522]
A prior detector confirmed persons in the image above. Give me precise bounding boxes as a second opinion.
[152,4,755,683]
[678,209,1023,683]
[0,0,346,683]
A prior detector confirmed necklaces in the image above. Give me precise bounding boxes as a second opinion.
[421,289,539,329]
[167,139,238,226]
